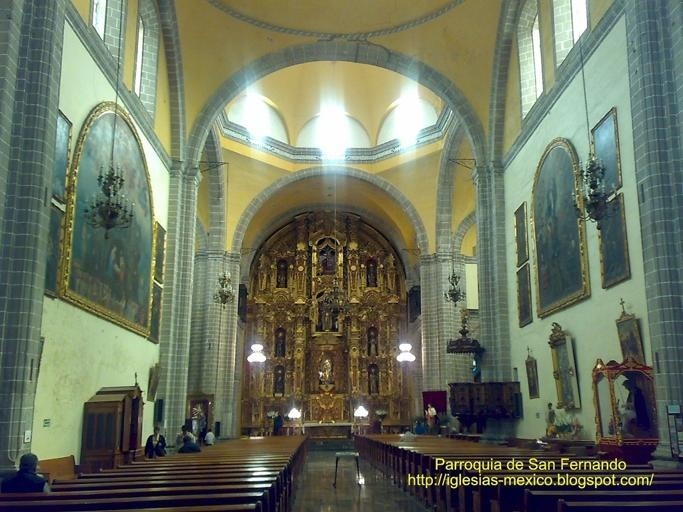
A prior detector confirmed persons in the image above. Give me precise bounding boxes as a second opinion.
[368,332,377,356]
[616,380,649,431]
[178,435,202,454]
[321,302,333,331]
[275,331,283,355]
[535,176,566,299]
[369,366,378,393]
[543,402,556,436]
[272,412,283,435]
[198,426,205,439]
[176,424,195,444]
[275,369,283,393]
[414,403,437,434]
[143,427,166,458]
[0,452,53,494]
[203,429,215,447]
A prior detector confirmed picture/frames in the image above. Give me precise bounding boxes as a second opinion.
[43,102,166,343]
[548,322,581,410]
[615,312,646,367]
[525,355,539,399]
[512,107,631,328]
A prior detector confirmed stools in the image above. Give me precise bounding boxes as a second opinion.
[333,451,360,486]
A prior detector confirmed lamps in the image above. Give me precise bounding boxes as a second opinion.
[443,184,466,307]
[212,250,235,309]
[570,2,623,229]
[83,1,134,240]
